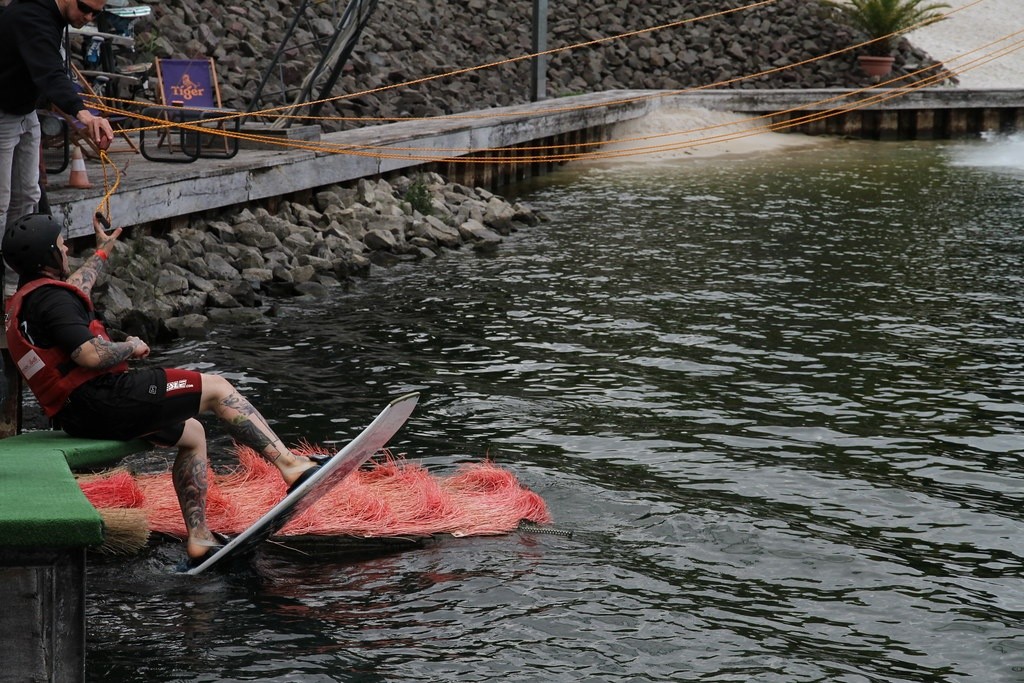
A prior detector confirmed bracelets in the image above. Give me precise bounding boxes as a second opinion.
[95,250,106,260]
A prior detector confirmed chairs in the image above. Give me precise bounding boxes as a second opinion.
[139,57,241,164]
[36,81,129,174]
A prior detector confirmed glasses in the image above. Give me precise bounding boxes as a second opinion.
[76,0,103,18]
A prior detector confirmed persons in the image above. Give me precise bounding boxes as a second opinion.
[0,0,115,251]
[1,214,332,566]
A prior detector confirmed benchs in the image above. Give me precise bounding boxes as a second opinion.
[0,429,156,547]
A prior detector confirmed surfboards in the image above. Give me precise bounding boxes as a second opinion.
[188,389,423,577]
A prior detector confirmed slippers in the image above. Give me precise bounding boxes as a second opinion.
[286,454,332,495]
[186,531,232,569]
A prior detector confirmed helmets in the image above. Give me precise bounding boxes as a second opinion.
[1,212,62,280]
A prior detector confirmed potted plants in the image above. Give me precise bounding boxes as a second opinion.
[825,0,954,79]
